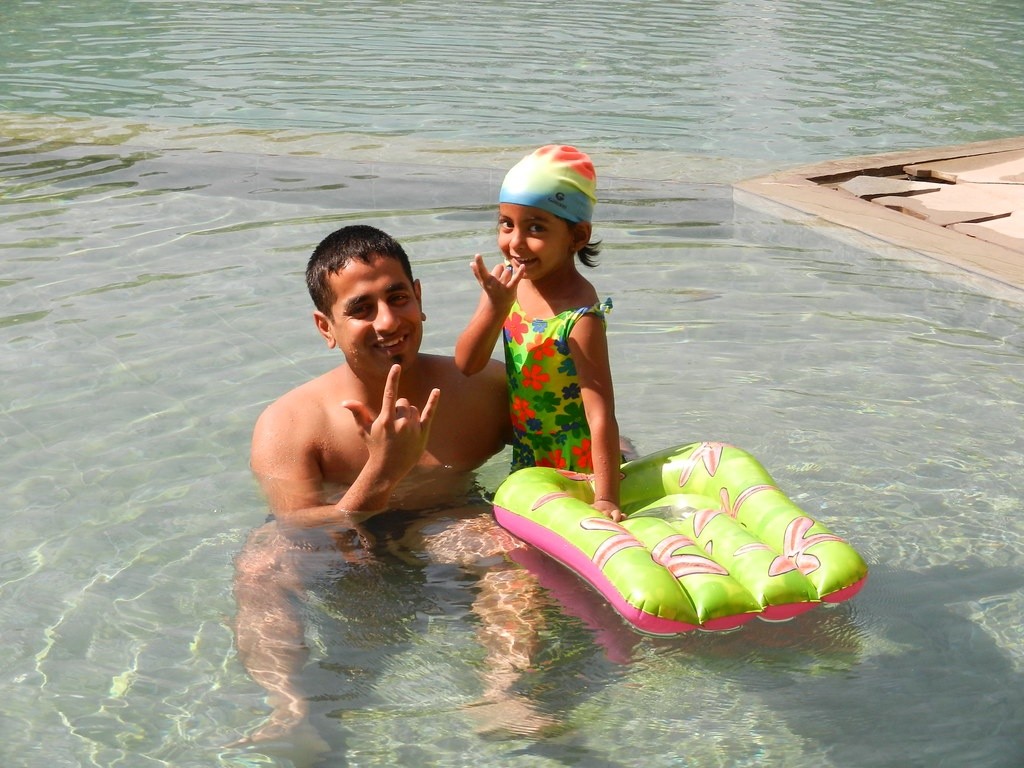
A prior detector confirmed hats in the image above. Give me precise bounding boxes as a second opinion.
[498,143,597,224]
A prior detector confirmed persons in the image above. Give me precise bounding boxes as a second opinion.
[454,144,633,527]
[230,225,559,745]
